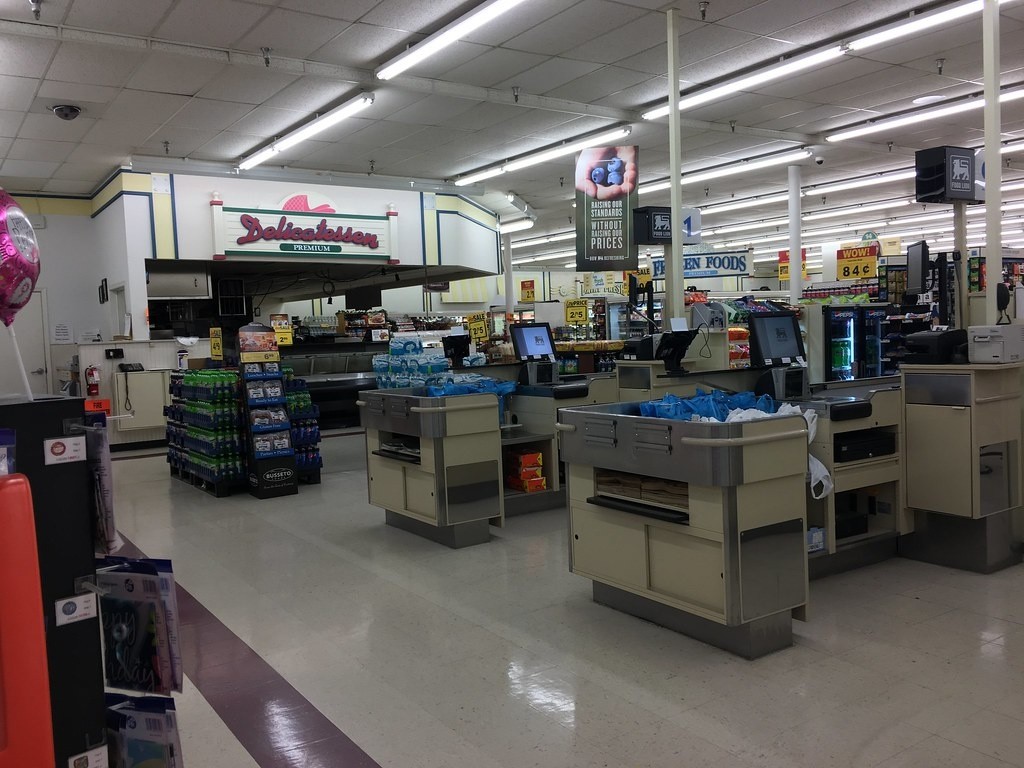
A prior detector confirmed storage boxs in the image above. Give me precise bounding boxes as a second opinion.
[506,447,546,493]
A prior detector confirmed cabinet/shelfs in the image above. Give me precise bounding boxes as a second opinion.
[799,256,1024,327]
[301,308,469,340]
[586,292,664,343]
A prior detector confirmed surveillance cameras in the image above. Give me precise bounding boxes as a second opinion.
[815,157,824,165]
[52,105,81,120]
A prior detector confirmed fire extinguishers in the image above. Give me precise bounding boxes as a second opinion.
[85,364,103,396]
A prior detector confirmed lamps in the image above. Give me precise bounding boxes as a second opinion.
[454,125,633,187]
[374,0,524,82]
[638,148,814,194]
[637,0,1014,122]
[824,84,1024,143]
[508,179,1024,270]
[238,92,376,171]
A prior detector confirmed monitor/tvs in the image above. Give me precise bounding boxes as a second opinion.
[444,334,470,358]
[654,330,699,361]
[628,274,638,314]
[906,240,929,295]
[747,311,807,368]
[509,322,557,362]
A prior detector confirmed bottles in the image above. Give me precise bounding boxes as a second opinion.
[832,341,852,381]
[372,331,521,432]
[618,303,663,338]
[550,298,606,342]
[594,344,637,372]
[163,349,324,495]
[556,352,579,374]
[412,315,462,330]
[303,309,368,337]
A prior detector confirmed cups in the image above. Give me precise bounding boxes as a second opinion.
[772,367,786,400]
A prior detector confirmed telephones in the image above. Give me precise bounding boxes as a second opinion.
[118,363,144,371]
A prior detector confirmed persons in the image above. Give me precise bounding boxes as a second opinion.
[575,145,637,200]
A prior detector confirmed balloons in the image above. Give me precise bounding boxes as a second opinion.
[0,186,40,327]
